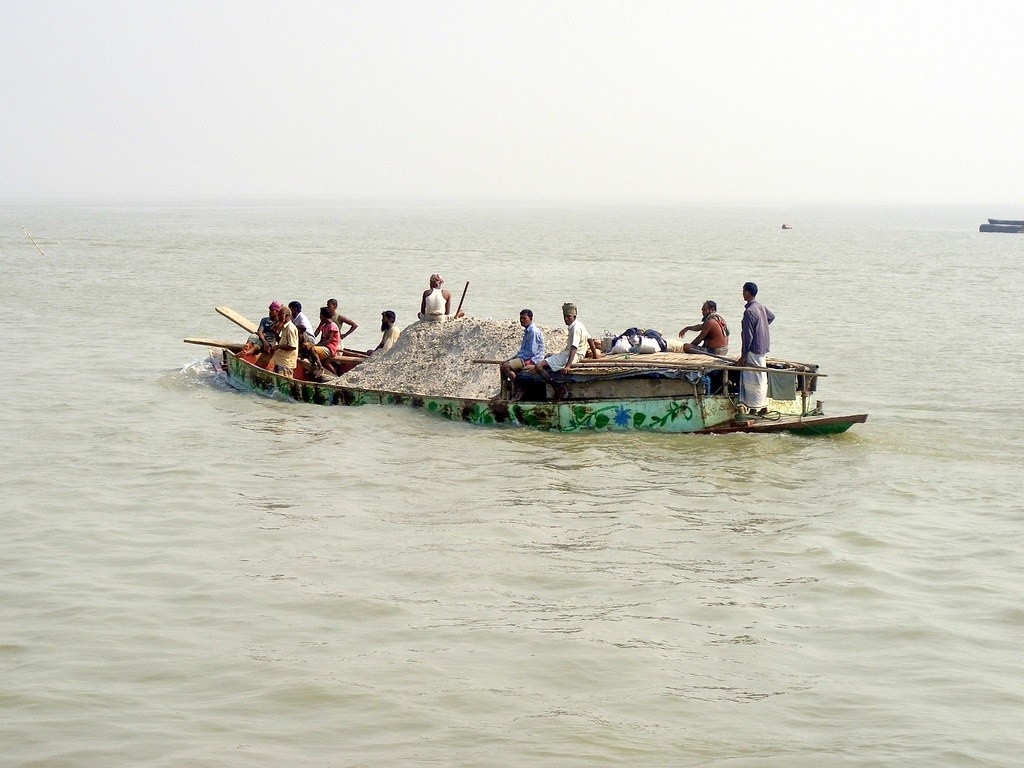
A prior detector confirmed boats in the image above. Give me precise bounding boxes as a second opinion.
[220,341,869,436]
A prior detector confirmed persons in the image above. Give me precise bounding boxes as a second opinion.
[305,299,358,378]
[270,306,298,379]
[364,311,401,356]
[234,300,281,357]
[737,282,776,415]
[489,309,545,402]
[679,301,730,356]
[418,274,464,322]
[288,302,315,357]
[535,302,604,401]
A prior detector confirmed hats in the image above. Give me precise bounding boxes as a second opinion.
[561,301,578,317]
[268,301,284,311]
[430,274,445,284]
